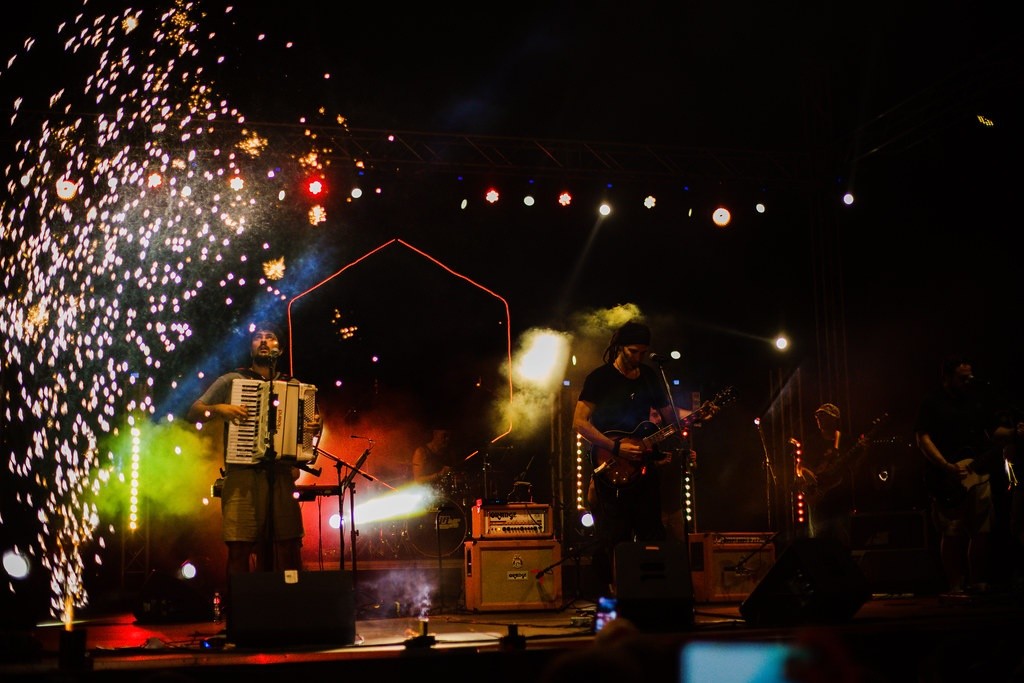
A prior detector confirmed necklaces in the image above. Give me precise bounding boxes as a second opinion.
[615,358,638,400]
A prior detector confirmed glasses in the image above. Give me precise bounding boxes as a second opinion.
[815,413,831,418]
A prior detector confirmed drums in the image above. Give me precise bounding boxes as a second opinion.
[480,446,517,473]
[436,471,478,500]
[403,496,468,558]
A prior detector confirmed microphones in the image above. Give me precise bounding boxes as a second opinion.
[649,353,670,363]
[536,571,544,579]
[268,348,280,359]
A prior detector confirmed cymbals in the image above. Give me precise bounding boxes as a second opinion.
[388,456,421,467]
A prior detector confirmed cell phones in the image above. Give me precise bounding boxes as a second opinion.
[677,641,815,683]
[594,597,623,633]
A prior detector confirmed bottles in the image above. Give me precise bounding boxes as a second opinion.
[213,593,222,624]
[597,599,617,630]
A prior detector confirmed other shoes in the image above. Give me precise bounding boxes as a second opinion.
[591,605,611,630]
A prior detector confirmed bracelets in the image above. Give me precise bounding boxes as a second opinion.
[612,438,620,457]
[943,462,948,470]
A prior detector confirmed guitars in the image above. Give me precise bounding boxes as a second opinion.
[794,407,893,504]
[933,418,1023,509]
[589,387,739,484]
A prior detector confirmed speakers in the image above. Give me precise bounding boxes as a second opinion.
[738,536,876,629]
[848,512,933,582]
[849,444,925,513]
[128,570,215,623]
[612,540,695,634]
[463,539,562,612]
[222,569,357,647]
[688,532,776,604]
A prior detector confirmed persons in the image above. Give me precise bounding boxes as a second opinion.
[188,323,323,652]
[913,361,1024,600]
[794,403,872,549]
[412,419,457,485]
[573,321,721,634]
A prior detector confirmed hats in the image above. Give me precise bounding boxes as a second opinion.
[814,404,841,419]
[617,319,651,346]
[251,321,286,349]
